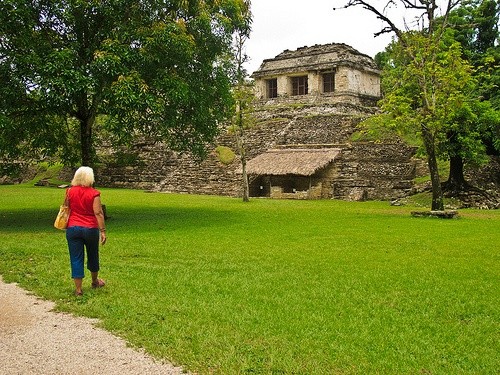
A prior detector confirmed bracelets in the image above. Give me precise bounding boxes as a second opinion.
[100,228,106,232]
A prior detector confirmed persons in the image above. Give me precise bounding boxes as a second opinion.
[63,166,108,296]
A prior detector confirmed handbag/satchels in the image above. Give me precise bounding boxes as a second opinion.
[53,187,72,231]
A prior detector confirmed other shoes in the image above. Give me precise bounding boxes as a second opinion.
[75,288,82,297]
[92,280,105,287]
